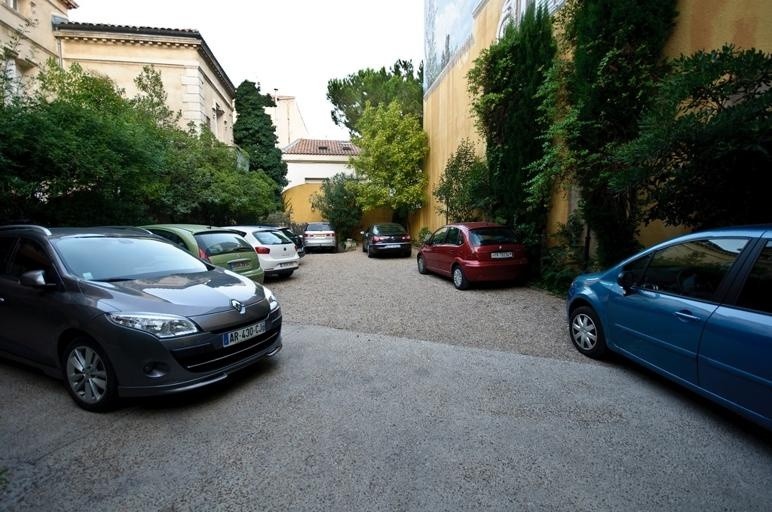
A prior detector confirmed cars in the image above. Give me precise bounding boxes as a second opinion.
[359,223,412,258]
[417,219,527,289]
[0,222,283,416]
[134,221,338,289]
[565,223,771,427]
